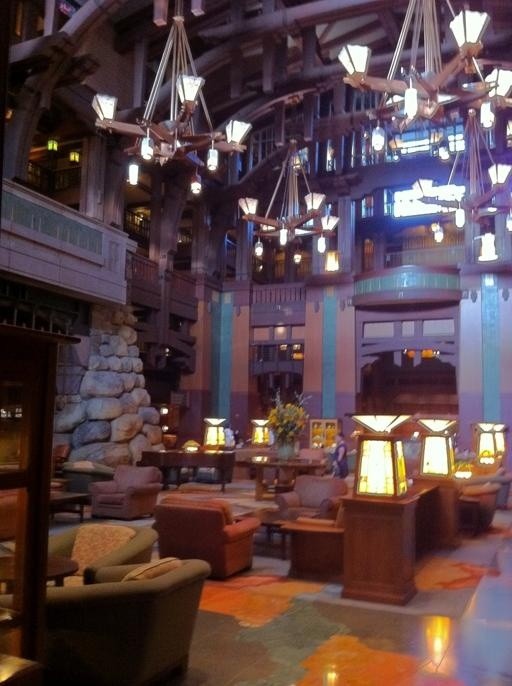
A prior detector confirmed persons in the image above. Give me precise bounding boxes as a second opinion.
[319,442,358,478]
[331,431,349,478]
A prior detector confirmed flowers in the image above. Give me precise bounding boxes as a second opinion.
[270,404,309,443]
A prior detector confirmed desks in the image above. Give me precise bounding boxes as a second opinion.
[142,451,235,491]
[250,456,328,502]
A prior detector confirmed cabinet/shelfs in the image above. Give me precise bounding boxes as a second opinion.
[0,323,81,661]
[308,418,339,448]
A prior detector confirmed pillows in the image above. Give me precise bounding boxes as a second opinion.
[121,557,182,580]
[70,525,137,576]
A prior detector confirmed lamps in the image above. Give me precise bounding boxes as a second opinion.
[48,140,58,152]
[69,151,80,164]
[205,419,226,445]
[473,421,507,465]
[417,417,465,479]
[252,419,270,444]
[339,415,419,498]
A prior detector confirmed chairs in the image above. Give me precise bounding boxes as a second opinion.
[282,503,346,582]
[49,559,212,686]
[0,653,43,686]
[49,522,159,585]
[89,466,163,519]
[275,475,348,520]
[153,496,261,576]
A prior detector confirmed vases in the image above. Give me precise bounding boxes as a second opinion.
[278,443,295,459]
